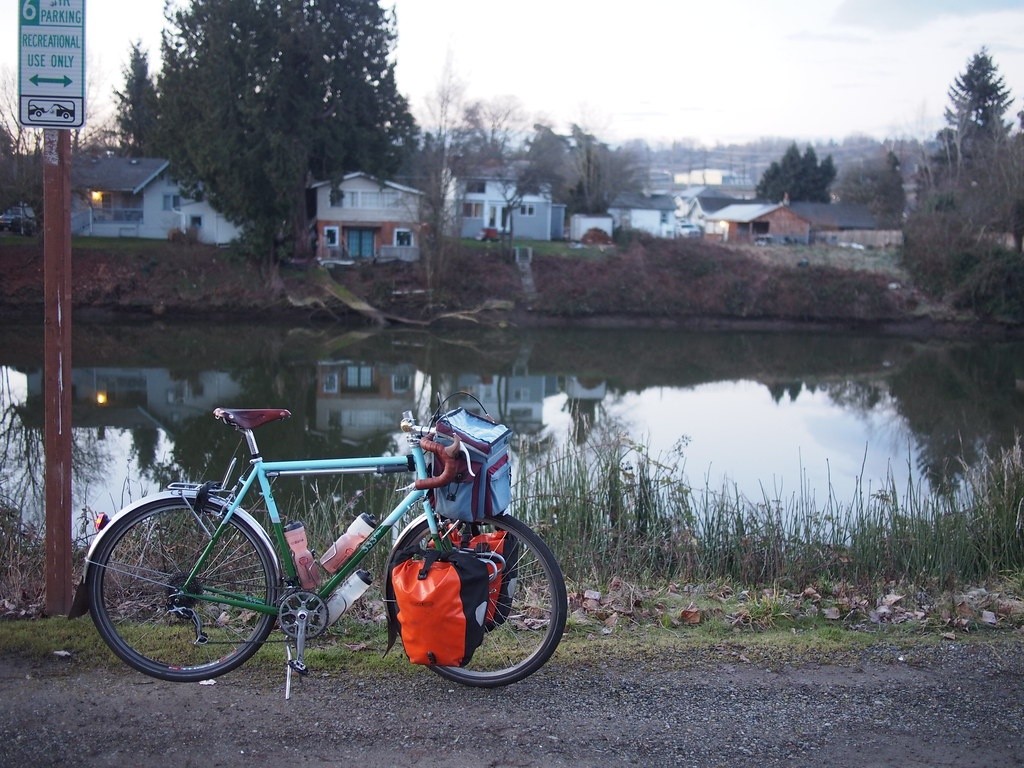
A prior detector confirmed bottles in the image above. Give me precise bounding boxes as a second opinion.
[319,512,377,575]
[284,519,319,589]
[319,569,373,627]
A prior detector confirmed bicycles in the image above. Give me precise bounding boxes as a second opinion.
[67,408,567,701]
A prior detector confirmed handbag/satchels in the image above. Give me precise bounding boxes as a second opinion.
[433,408,512,522]
[389,541,488,668]
[427,526,516,634]
